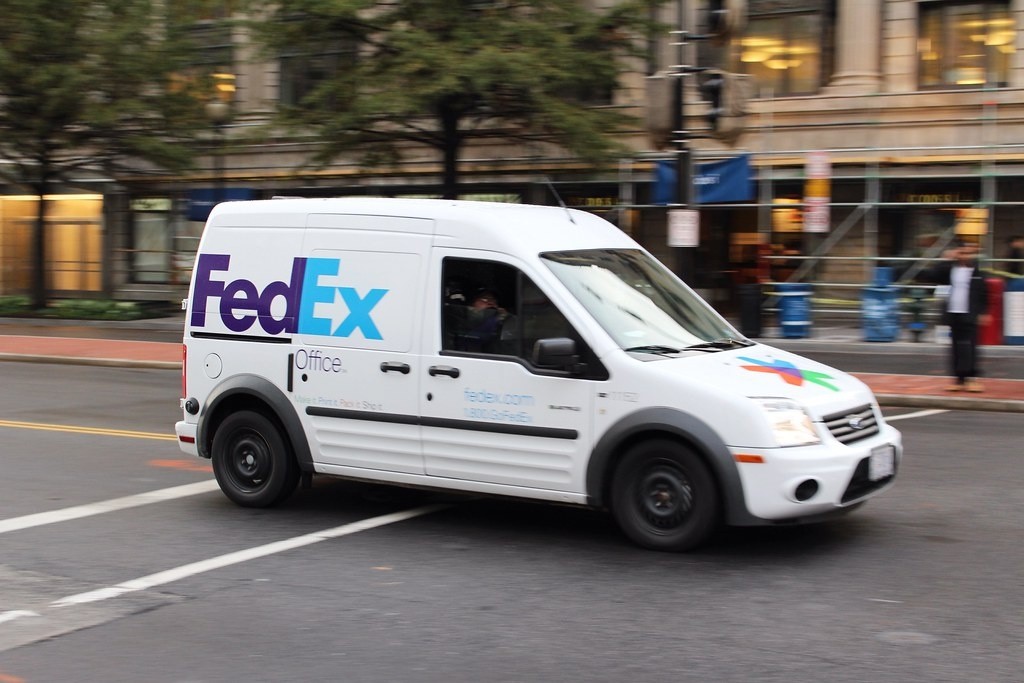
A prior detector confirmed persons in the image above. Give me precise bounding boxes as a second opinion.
[930,241,992,393]
[452,279,522,356]
[1002,235,1024,276]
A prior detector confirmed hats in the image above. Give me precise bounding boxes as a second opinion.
[471,283,499,298]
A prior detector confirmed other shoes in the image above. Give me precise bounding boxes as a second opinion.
[965,382,985,391]
[944,384,963,390]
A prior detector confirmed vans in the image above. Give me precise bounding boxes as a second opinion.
[169,197,905,554]
